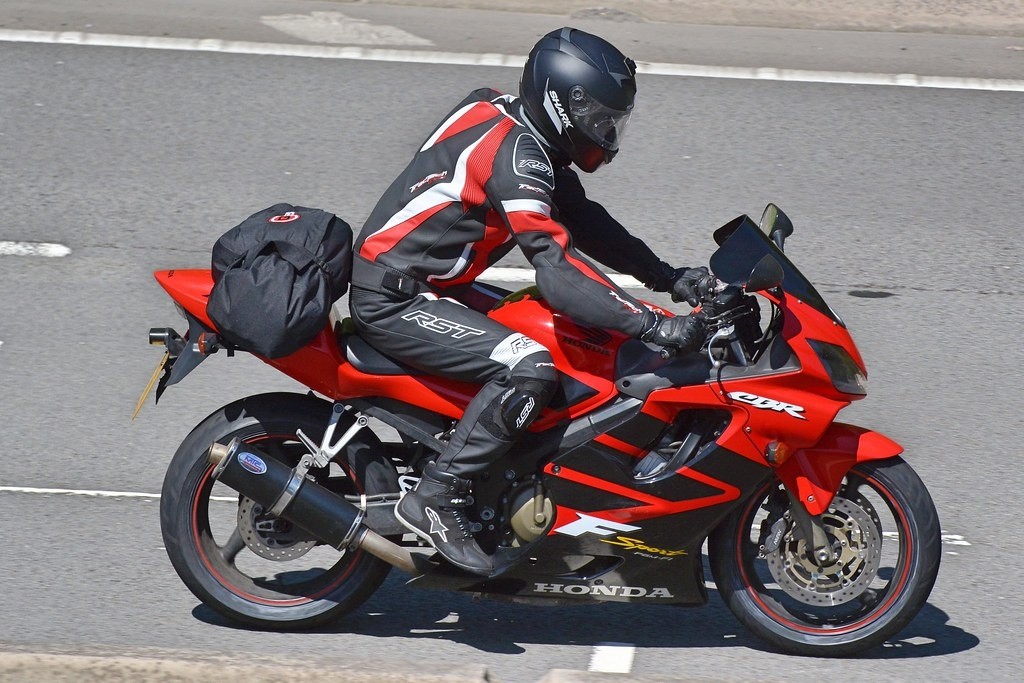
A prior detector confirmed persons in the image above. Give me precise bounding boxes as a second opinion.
[347,26,718,576]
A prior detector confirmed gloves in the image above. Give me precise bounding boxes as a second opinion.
[639,314,705,356]
[647,263,711,308]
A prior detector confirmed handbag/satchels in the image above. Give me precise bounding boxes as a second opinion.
[206,203,354,360]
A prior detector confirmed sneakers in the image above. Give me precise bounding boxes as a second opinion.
[394,461,494,576]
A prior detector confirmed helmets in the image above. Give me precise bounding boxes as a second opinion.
[521,28,638,172]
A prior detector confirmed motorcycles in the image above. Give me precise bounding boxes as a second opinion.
[131,202,941,658]
